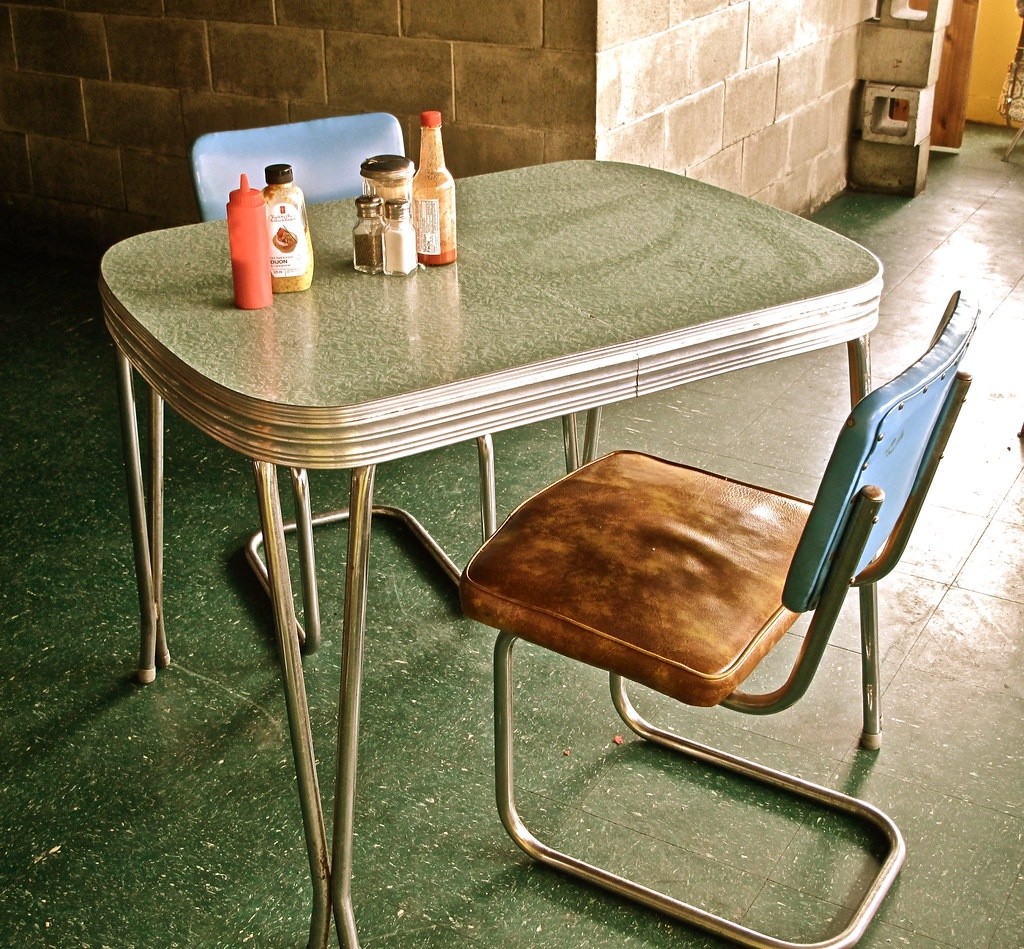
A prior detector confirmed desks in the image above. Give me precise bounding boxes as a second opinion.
[98,162,887,949]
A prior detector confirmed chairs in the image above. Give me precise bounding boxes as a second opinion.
[460,291,1000,949]
[192,109,499,651]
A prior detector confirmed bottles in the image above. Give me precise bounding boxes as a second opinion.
[260,163,314,294]
[225,173,274,310]
[359,154,416,227]
[351,194,388,275]
[413,110,458,265]
[381,198,419,277]
